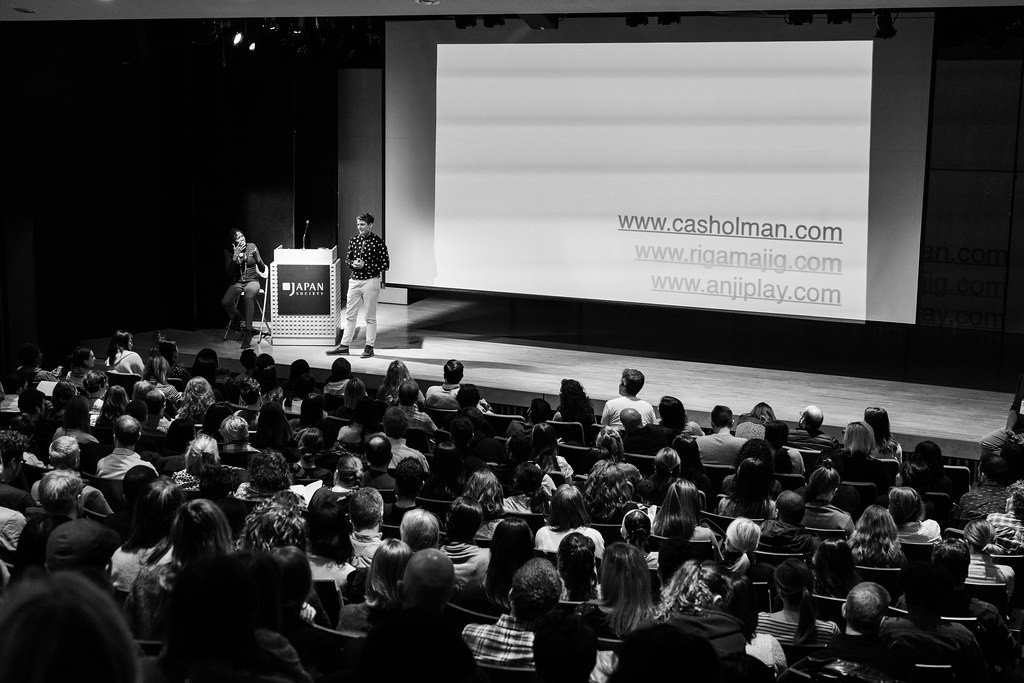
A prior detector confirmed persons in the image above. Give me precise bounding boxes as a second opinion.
[1,327,1024,683]
[219,228,266,349]
[325,212,388,358]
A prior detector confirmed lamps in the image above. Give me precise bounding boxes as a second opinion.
[828,11,852,25]
[482,13,505,28]
[658,11,682,26]
[873,13,899,41]
[455,14,478,30]
[784,9,813,25]
[625,14,648,28]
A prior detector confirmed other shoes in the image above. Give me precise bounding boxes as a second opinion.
[362,348,374,357]
[241,335,251,348]
[326,348,349,355]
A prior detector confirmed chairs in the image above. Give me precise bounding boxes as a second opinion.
[223,264,270,343]
[0,373,1024,683]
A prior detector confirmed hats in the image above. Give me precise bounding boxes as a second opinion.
[47,519,120,573]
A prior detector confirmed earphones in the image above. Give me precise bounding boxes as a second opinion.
[370,225,373,227]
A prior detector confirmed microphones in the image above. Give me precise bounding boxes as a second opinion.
[303,220,310,249]
